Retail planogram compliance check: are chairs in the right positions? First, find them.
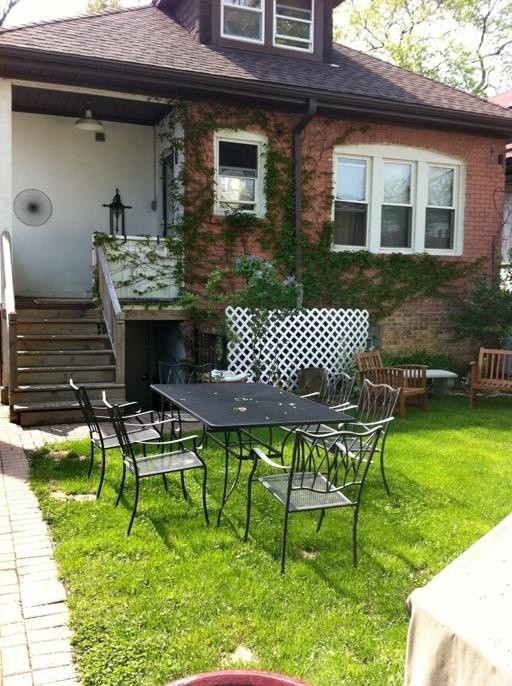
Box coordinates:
[279,369,354,472]
[356,352,429,419]
[69,379,168,499]
[158,361,212,449]
[296,379,401,496]
[100,391,209,536]
[243,426,380,574]
[469,347,512,407]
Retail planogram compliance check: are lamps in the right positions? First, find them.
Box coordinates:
[74,99,105,129]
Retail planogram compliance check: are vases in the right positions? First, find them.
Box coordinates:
[464,386,469,393]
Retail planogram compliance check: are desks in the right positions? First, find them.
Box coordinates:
[151,384,355,527]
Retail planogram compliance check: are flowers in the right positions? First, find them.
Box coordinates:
[460,370,473,385]
